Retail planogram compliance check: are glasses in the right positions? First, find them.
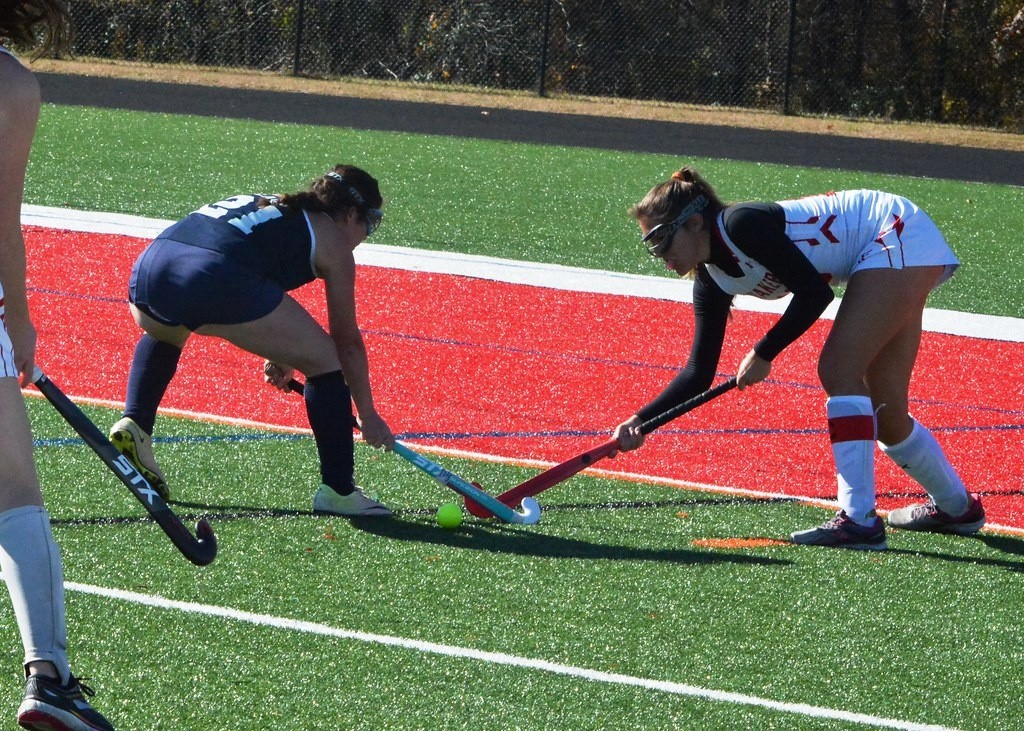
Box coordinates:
[364,210,383,235]
[641,222,676,258]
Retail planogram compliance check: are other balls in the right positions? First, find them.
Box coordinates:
[435,502,463,528]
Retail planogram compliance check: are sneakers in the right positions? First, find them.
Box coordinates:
[107,417,169,502]
[789,510,887,551]
[313,484,393,518]
[17,672,115,731]
[887,492,985,532]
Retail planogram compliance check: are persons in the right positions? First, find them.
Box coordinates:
[108,163,398,517]
[610,164,986,551]
[1,0,117,730]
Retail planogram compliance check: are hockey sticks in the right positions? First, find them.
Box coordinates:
[265,363,541,525]
[30,365,217,567]
[465,376,737,518]
